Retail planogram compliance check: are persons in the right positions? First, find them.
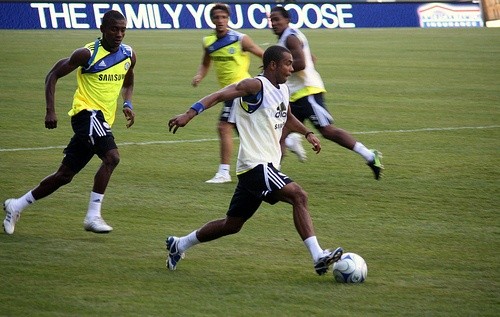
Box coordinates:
[2,10,137,236]
[165,3,385,276]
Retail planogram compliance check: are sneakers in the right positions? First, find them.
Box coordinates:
[287,132,307,162]
[366,148,385,180]
[83,216,114,233]
[2,198,21,234]
[205,170,232,184]
[313,246,344,276]
[165,235,182,271]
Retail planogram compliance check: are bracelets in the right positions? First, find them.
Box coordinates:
[190,101,206,115]
[122,100,133,110]
[305,130,314,139]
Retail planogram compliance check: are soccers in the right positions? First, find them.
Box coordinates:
[333,252,368,283]
